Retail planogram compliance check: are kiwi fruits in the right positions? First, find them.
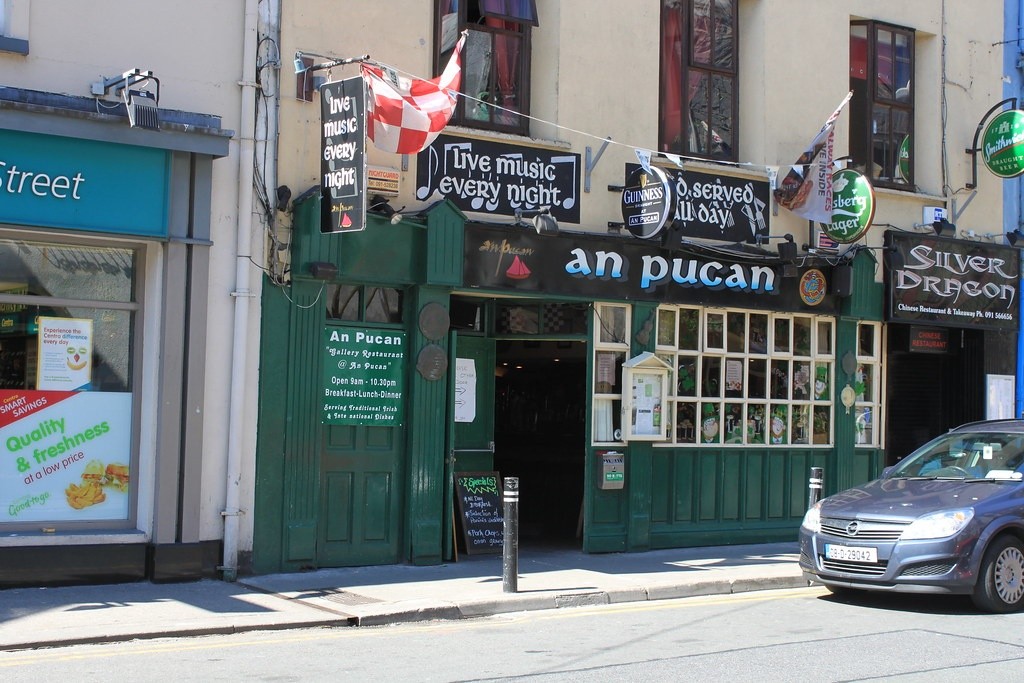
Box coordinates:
[67,347,87,353]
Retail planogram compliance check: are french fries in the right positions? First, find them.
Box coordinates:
[63,483,105,509]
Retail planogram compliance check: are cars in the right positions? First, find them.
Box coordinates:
[678,331,809,402]
[799,420,1024,614]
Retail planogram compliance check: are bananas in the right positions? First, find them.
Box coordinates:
[67,357,86,370]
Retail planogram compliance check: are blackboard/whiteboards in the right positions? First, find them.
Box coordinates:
[452,470,505,555]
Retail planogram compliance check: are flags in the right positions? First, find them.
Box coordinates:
[364,34,466,154]
[774,103,840,225]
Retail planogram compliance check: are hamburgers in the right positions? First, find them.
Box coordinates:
[80,461,129,491]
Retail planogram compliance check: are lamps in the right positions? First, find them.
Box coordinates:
[988,231,1017,247]
[914,218,957,238]
[656,218,686,251]
[104,69,162,130]
[370,196,402,224]
[514,208,560,236]
[855,243,905,271]
[755,234,798,261]
[309,262,338,281]
